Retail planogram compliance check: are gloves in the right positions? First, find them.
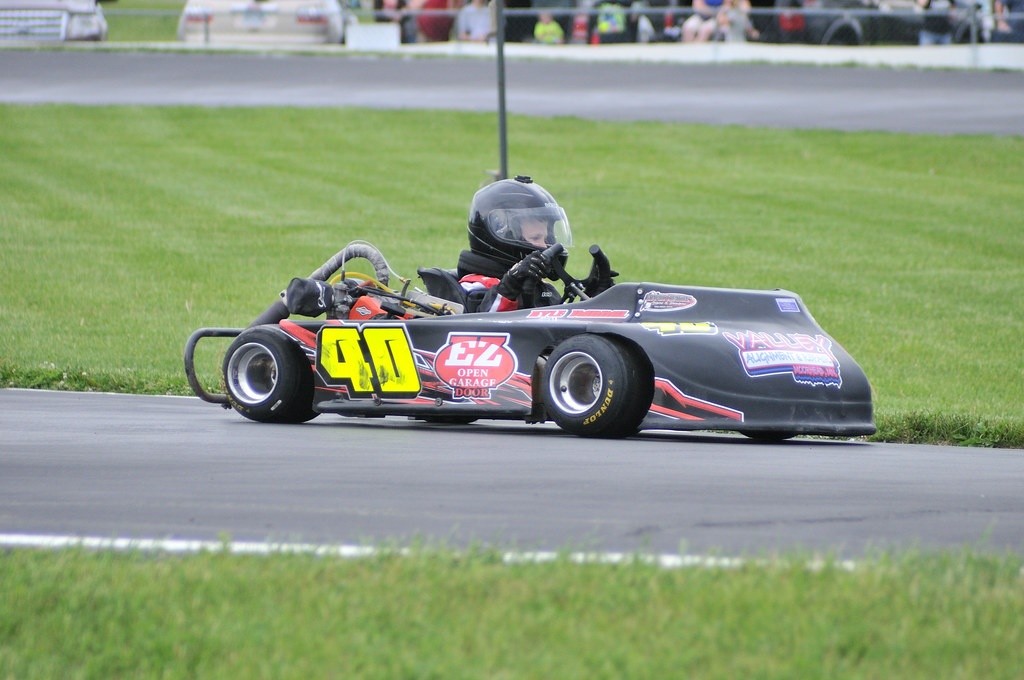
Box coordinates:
[496,250,550,301]
[610,270,619,287]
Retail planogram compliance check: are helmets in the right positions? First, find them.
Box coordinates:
[468,176,570,281]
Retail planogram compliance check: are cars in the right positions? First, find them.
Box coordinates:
[177,1,345,47]
[1,0,107,43]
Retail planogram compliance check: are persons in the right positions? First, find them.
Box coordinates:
[780,5,806,45]
[718,1,760,43]
[458,178,571,316]
[374,0,461,43]
[534,12,565,44]
[916,0,1013,45]
[457,0,506,44]
[680,0,726,43]
[628,0,685,42]
[594,0,631,44]
